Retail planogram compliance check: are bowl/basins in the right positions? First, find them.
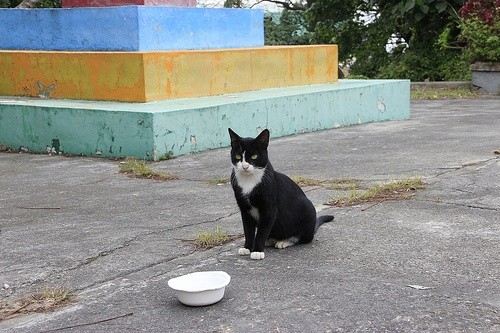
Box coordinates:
[167,270,231,306]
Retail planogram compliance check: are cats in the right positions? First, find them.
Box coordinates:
[228,127,335,259]
